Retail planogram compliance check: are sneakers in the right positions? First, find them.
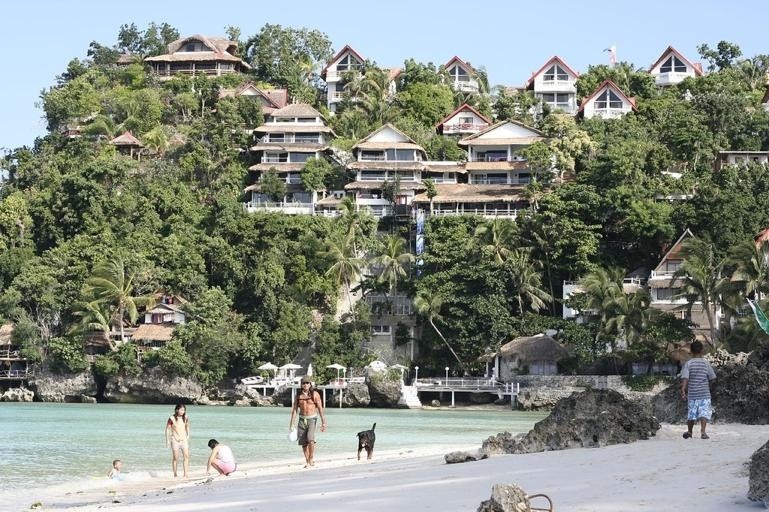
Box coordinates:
[683,431,693,438]
[701,433,709,439]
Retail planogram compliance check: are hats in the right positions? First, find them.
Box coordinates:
[302,376,310,384]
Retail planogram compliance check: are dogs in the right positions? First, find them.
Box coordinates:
[355,422,377,461]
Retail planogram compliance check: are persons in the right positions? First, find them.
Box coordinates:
[107,459,123,478]
[677,341,717,439]
[164,403,191,480]
[206,438,236,476]
[290,375,325,468]
